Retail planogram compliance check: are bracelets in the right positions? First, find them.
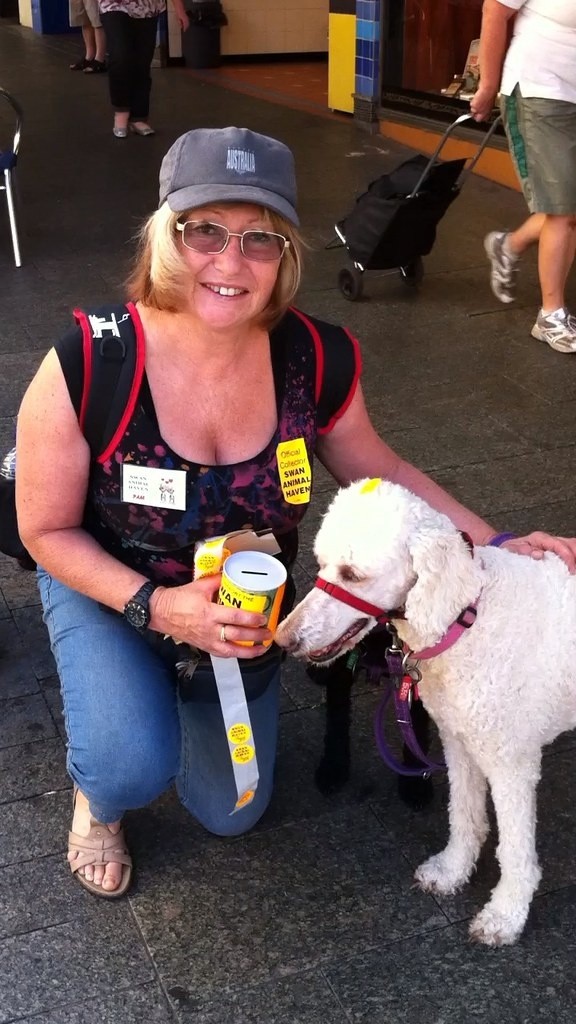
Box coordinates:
[489,533,515,546]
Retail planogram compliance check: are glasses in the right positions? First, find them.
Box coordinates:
[174,219,290,262]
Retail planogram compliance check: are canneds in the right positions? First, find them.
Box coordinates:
[216,551,287,652]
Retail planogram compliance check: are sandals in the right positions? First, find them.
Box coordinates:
[69,56,106,74]
[67,781,133,899]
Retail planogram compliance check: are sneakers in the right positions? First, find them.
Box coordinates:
[482,230,524,304]
[530,303,576,353]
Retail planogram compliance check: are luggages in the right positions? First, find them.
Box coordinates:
[322,107,501,302]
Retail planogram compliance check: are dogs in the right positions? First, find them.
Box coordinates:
[274,478,575,948]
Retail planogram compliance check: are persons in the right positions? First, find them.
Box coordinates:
[98,0,189,138]
[15,126,576,895]
[69,0,106,72]
[471,0,576,353]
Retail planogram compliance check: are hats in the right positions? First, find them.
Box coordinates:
[157,126,300,228]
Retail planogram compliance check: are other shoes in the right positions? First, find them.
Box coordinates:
[113,127,128,138]
[129,124,155,136]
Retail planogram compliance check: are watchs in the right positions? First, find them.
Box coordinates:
[124,583,156,635]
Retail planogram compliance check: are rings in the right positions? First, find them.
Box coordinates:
[220,624,226,643]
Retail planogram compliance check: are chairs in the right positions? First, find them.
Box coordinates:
[0,85,25,267]
